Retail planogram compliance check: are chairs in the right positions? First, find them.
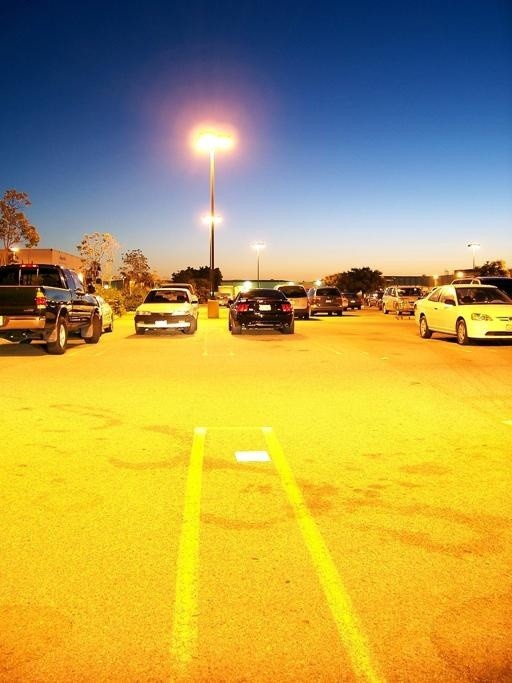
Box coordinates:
[461,290,486,302]
[153,295,185,302]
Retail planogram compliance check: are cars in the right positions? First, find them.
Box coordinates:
[229,288,295,336]
[307,286,342,316]
[95,294,114,334]
[135,281,199,336]
[365,291,385,309]
[341,293,361,311]
[413,277,512,345]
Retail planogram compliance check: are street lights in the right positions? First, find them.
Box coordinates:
[249,240,266,287]
[193,126,230,299]
[468,242,482,271]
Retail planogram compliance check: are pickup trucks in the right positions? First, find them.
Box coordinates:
[0,264,100,354]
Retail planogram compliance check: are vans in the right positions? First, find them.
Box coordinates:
[275,283,309,320]
[382,284,424,316]
